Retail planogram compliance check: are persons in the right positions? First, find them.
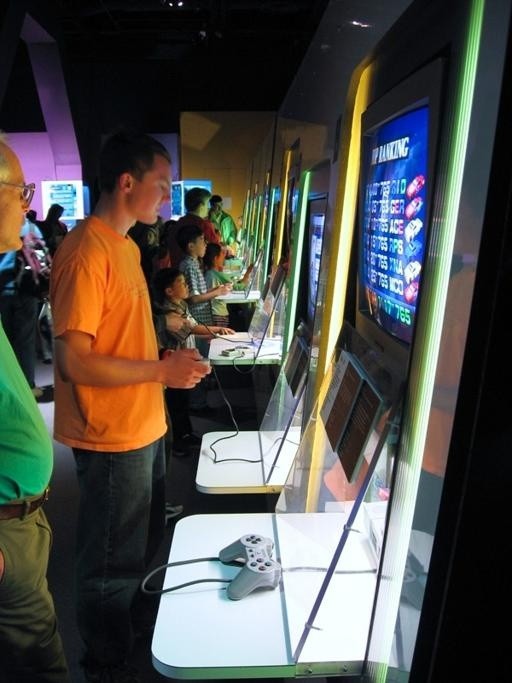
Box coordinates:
[0,139,72,682]
[49,126,211,681]
[1,185,263,454]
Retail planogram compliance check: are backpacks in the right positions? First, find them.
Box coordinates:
[15,223,55,300]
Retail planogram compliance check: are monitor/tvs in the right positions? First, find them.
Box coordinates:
[262,182,300,258]
[305,198,328,332]
[355,56,448,385]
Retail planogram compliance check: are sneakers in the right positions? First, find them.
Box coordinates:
[171,433,203,458]
[34,383,56,405]
[165,500,183,520]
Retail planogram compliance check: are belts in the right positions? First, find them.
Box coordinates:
[0,485,51,522]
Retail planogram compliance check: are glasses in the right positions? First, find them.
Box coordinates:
[1,181,36,206]
